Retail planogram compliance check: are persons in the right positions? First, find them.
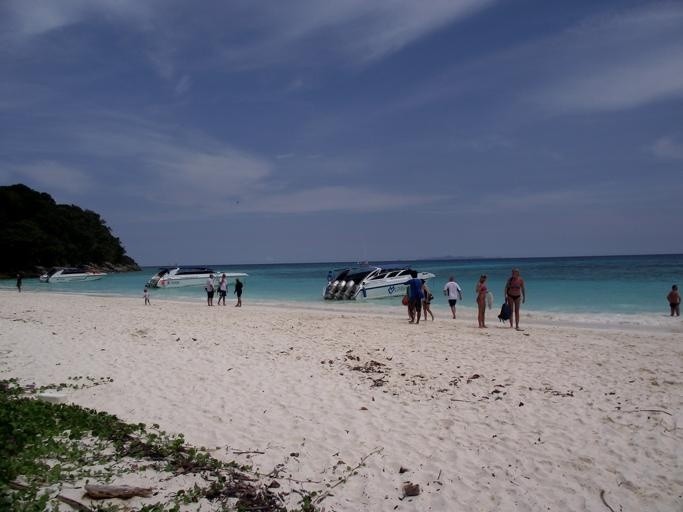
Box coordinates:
[15,273,22,292]
[666,284,681,316]
[404,270,425,323]
[420,279,434,321]
[406,287,416,320]
[206,274,215,305]
[141,287,149,304]
[443,276,462,319]
[233,278,242,306]
[216,273,228,306]
[504,269,525,328]
[326,270,333,285]
[475,273,489,328]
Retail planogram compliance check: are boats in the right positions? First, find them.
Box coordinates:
[143,264,252,289]
[320,265,438,306]
[39,266,109,284]
